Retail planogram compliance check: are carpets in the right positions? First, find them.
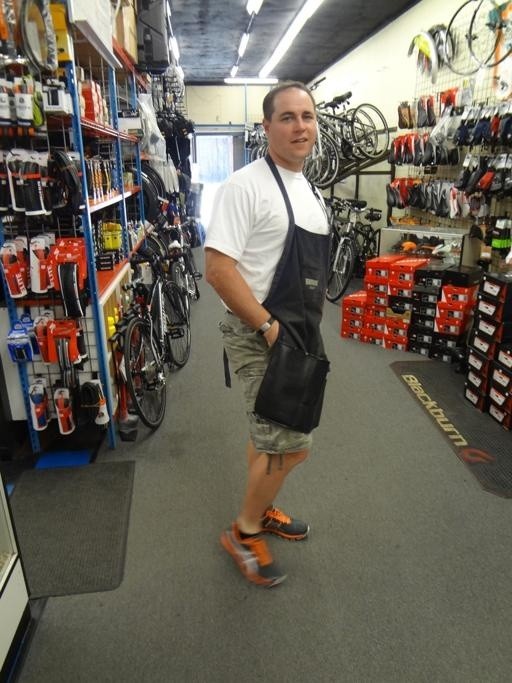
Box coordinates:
[384,355,511,498]
[8,460,138,600]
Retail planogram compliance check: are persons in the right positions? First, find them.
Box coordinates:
[202,81,334,588]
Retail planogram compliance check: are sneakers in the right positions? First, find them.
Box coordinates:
[218,520,287,588]
[260,503,311,540]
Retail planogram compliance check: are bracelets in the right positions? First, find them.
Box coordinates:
[259,313,275,336]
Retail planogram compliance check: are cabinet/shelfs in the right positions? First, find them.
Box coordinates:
[1,0,191,455]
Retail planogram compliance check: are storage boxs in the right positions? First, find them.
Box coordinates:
[339,255,511,430]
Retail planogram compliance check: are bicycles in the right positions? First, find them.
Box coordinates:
[322,194,382,302]
[123,221,202,429]
[244,79,389,188]
